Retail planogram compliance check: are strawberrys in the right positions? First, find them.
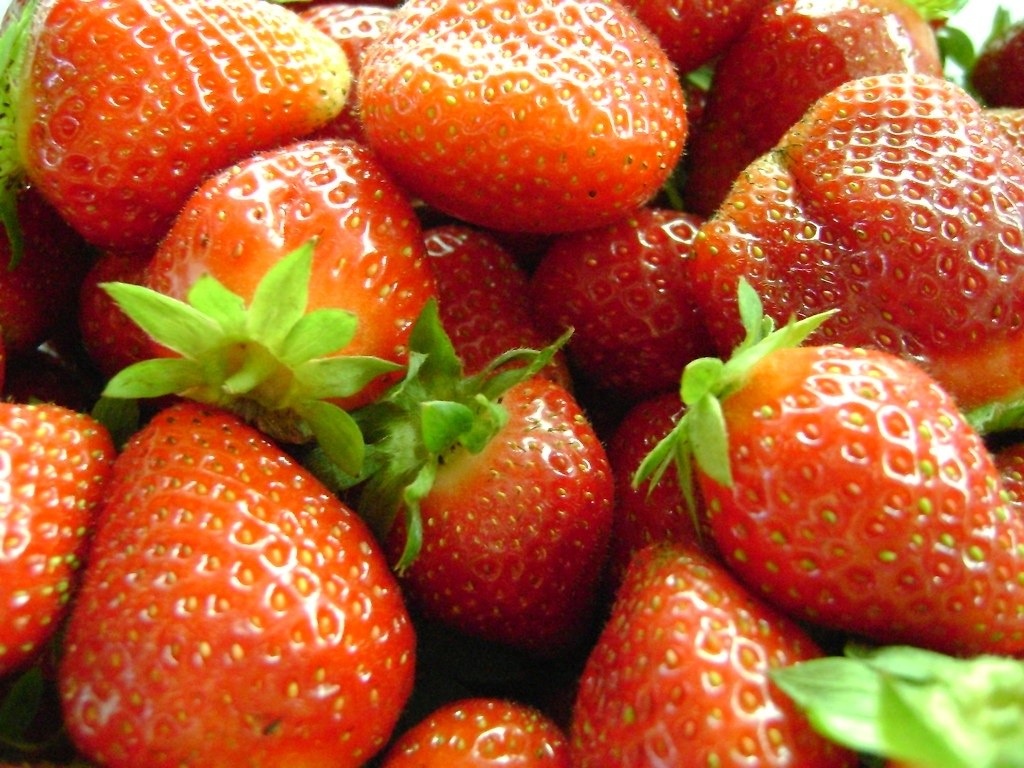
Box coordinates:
[0,0,1024,768]
